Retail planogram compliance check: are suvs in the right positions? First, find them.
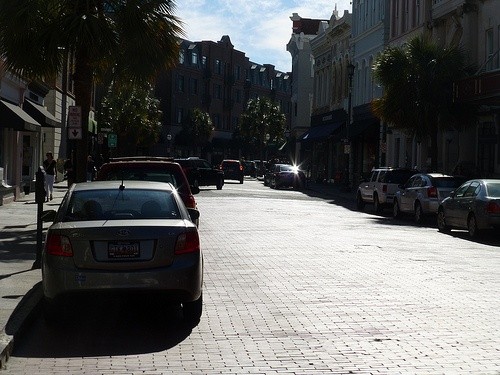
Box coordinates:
[219,159,247,184]
[90,155,201,229]
[173,156,225,191]
[354,165,419,215]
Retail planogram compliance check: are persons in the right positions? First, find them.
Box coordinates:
[88,156,95,182]
[368,158,378,173]
[241,157,247,169]
[269,157,277,167]
[99,154,104,164]
[55,158,63,182]
[43,151,58,202]
[250,162,257,178]
[279,157,288,163]
[421,152,438,173]
[405,159,412,169]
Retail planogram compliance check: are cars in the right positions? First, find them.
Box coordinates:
[391,172,469,227]
[436,178,500,240]
[40,178,205,328]
[241,157,306,191]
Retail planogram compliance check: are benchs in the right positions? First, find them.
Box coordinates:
[79,201,161,215]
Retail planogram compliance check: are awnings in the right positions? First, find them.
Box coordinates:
[24,98,61,127]
[297,120,346,142]
[0,100,41,131]
[334,115,380,146]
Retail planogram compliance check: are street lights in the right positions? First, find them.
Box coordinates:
[166,134,172,157]
[344,61,356,193]
[265,133,270,169]
